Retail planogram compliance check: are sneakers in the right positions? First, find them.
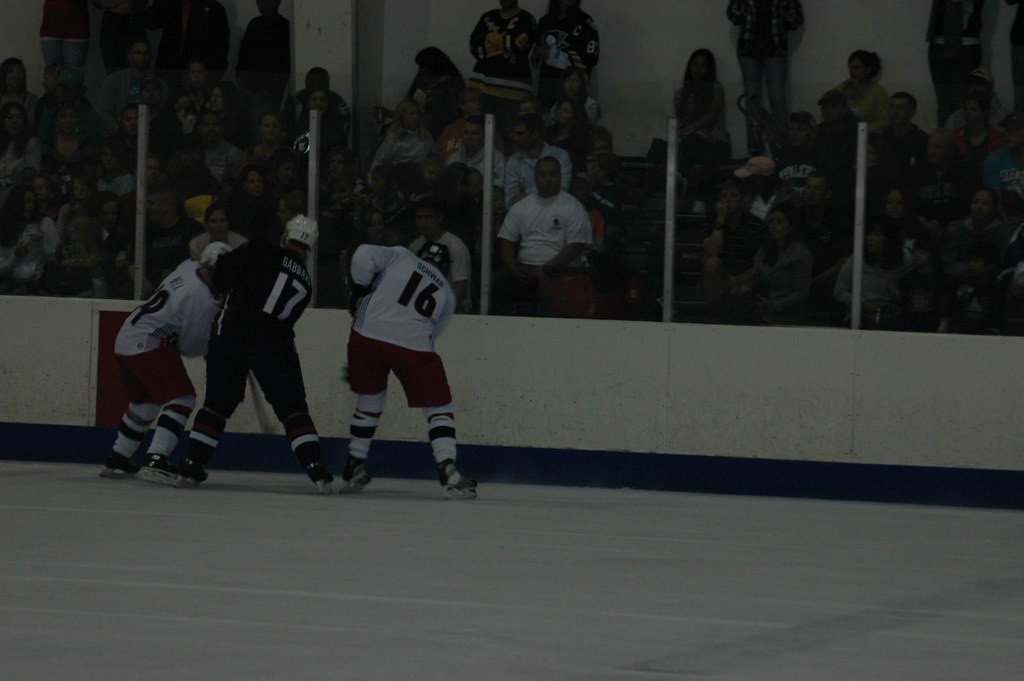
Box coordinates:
[99,448,129,479]
[134,453,177,485]
[303,458,334,496]
[339,453,373,493]
[437,458,478,499]
[173,458,208,488]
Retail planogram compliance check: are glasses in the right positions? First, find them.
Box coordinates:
[415,214,433,220]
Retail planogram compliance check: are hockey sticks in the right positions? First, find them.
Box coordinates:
[248,371,272,434]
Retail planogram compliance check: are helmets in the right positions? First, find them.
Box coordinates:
[280,214,319,252]
[200,241,234,268]
[417,240,450,270]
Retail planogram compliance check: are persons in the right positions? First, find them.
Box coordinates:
[91,0,151,75]
[406,203,472,313]
[925,0,984,128]
[674,48,731,203]
[234,0,290,82]
[186,204,248,261]
[530,0,600,109]
[726,0,805,164]
[177,214,335,494]
[465,0,537,126]
[0,36,1024,338]
[39,0,91,85]
[443,114,506,189]
[830,49,890,134]
[1004,0,1024,116]
[144,0,231,80]
[492,156,595,319]
[97,242,234,486]
[503,113,572,212]
[342,241,480,499]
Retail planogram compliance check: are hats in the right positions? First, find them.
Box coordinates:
[734,155,776,178]
